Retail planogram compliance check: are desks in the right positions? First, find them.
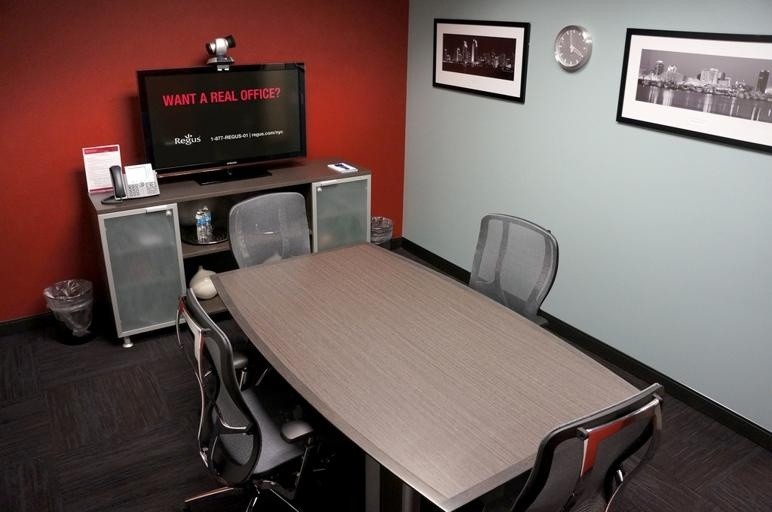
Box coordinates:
[211,241,643,512]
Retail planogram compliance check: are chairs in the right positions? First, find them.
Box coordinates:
[467,211,558,325]
[510,381,666,511]
[228,192,311,269]
[174,286,336,512]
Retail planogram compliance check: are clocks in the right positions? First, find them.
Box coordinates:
[552,24,593,71]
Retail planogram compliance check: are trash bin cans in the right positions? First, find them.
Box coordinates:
[43,278,95,346]
[371,216,393,251]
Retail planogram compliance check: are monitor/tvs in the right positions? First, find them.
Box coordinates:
[136,60,307,186]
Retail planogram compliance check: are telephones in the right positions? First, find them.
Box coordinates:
[101,162,161,204]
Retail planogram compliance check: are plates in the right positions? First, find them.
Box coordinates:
[180,224,228,244]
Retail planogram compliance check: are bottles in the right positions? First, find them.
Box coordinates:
[195,208,205,240]
[203,206,214,236]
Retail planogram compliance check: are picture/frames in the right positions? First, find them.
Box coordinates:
[432,17,530,104]
[616,27,772,156]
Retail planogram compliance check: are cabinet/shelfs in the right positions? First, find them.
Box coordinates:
[85,156,374,348]
